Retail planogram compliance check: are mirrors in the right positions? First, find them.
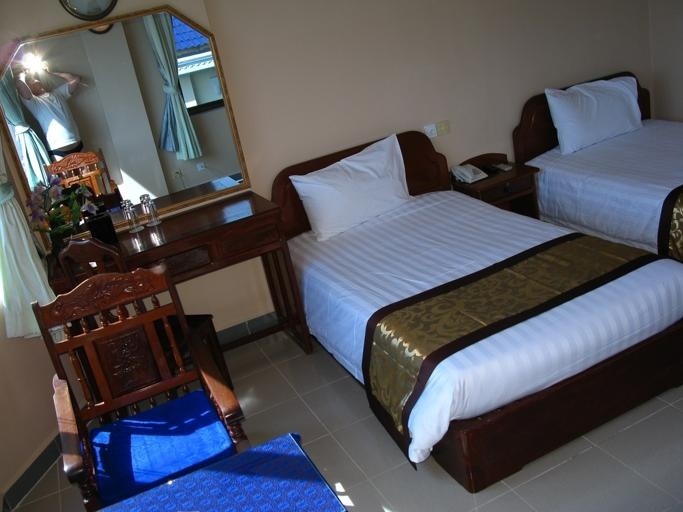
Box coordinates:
[1,6,254,261]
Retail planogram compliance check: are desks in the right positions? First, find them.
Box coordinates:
[43,191,313,425]
[106,170,244,224]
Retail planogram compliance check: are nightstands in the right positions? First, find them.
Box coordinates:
[451,147,541,221]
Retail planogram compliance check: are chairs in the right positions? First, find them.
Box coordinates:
[23,260,246,511]
[56,234,238,398]
[42,144,126,214]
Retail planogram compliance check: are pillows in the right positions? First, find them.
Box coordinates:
[541,75,639,156]
[287,131,413,242]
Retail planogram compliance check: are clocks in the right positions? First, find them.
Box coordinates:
[87,23,114,35]
[57,0,121,21]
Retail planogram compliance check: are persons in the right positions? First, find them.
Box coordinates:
[13,62,85,161]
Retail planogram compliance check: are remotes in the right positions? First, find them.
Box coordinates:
[493,163,512,171]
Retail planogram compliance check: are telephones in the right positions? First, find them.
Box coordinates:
[451,163,489,184]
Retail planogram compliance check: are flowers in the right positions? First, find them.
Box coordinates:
[26,175,101,237]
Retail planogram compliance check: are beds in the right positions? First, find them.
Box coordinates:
[266,126,681,493]
[511,71,682,259]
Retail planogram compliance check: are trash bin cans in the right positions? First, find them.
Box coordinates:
[147,314,235,401]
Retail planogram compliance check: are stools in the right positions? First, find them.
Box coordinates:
[97,431,349,510]
